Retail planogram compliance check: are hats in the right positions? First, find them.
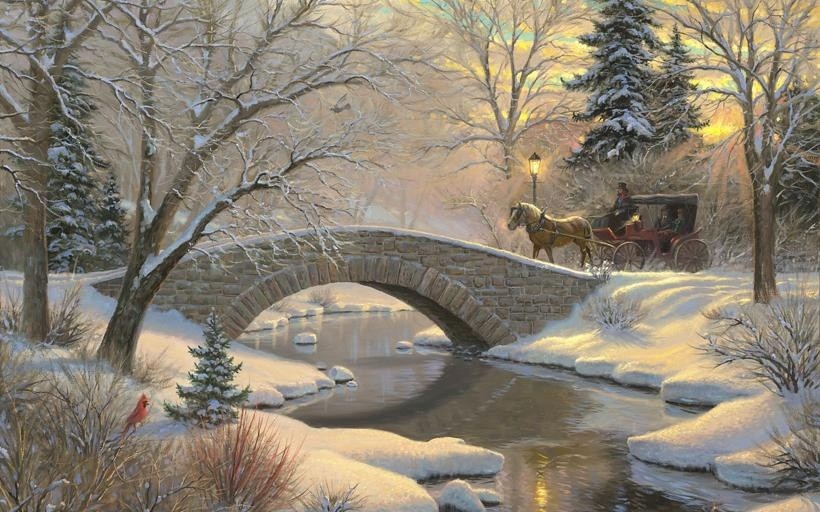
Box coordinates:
[616,181,626,189]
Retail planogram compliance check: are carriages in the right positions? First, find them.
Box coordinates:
[507,181,710,273]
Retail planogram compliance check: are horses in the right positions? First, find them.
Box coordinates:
[507,201,593,270]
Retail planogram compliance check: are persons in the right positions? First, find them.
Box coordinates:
[658,207,689,252]
[609,182,634,235]
[657,206,676,230]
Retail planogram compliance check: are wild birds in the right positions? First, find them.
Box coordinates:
[120,393,150,440]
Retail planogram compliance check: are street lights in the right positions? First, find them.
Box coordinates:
[528,151,541,206]
